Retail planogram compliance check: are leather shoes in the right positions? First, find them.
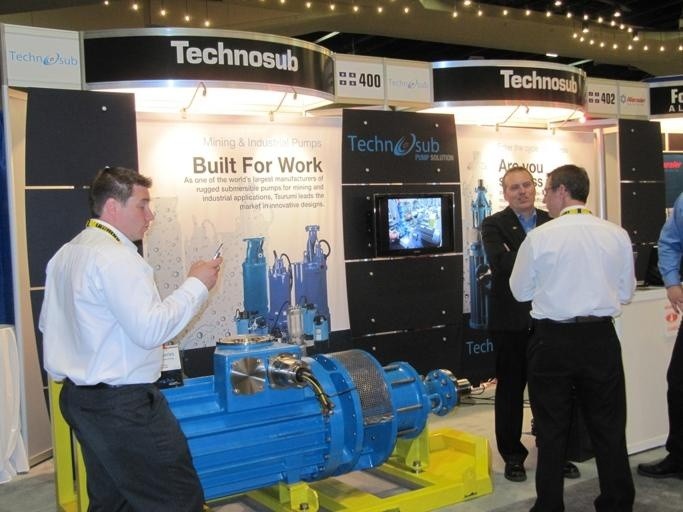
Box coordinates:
[503,464,527,482]
[637,460,683,479]
[563,462,580,480]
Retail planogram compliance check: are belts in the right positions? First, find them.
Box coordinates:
[67,378,127,390]
[550,316,611,324]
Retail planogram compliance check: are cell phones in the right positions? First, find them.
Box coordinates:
[213,244,223,261]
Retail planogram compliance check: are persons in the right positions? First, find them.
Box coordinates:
[481,167,581,482]
[638,192,682,479]
[510,164,637,511]
[39,165,223,512]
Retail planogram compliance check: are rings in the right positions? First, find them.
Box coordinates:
[216,266,220,271]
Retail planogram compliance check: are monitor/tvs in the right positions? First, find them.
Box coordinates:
[372,191,455,257]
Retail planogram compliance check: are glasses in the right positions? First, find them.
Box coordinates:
[542,185,560,196]
